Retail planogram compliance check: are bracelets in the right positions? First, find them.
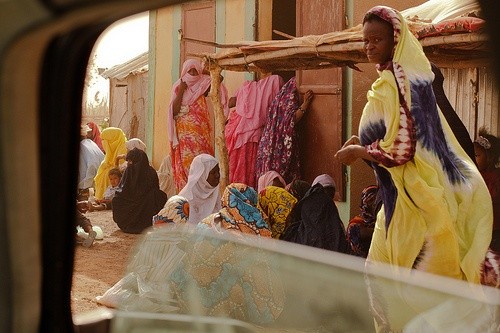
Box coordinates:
[299,106,305,115]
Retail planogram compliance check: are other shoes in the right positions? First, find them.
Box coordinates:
[74,234,86,244]
[83,231,97,248]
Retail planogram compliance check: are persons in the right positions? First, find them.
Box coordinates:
[77,122,382,258]
[169,57,224,196]
[223,75,313,189]
[472,127,500,252]
[334,6,493,285]
